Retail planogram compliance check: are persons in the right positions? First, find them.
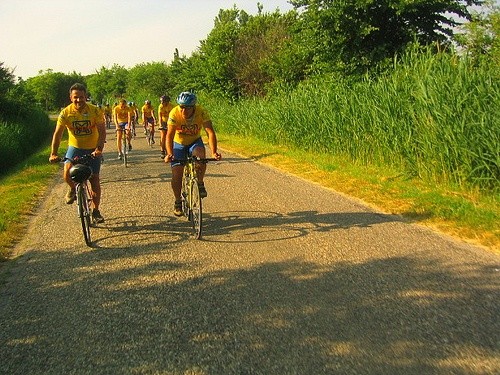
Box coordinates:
[142,100,157,144]
[112,102,117,124]
[164,92,221,217]
[99,105,104,113]
[128,101,137,136]
[104,103,112,124]
[158,96,177,159]
[114,99,132,160]
[132,102,139,124]
[49,83,106,223]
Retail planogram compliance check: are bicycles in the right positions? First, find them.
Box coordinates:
[161,151,220,240]
[115,126,131,168]
[106,114,167,158]
[59,152,102,245]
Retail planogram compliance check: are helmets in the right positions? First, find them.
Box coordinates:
[160,95,170,102]
[176,92,197,105]
[132,102,135,105]
[69,164,92,183]
[145,100,151,105]
[106,103,109,106]
[127,102,132,105]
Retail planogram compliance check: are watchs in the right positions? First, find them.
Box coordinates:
[96,146,103,151]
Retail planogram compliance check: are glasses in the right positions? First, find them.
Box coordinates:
[180,105,194,109]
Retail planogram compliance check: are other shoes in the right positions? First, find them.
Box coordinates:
[128,145,132,151]
[144,129,147,135]
[65,189,76,204]
[161,152,165,158]
[92,210,104,223]
[199,186,207,197]
[174,201,184,215]
[118,154,122,159]
[152,140,155,144]
